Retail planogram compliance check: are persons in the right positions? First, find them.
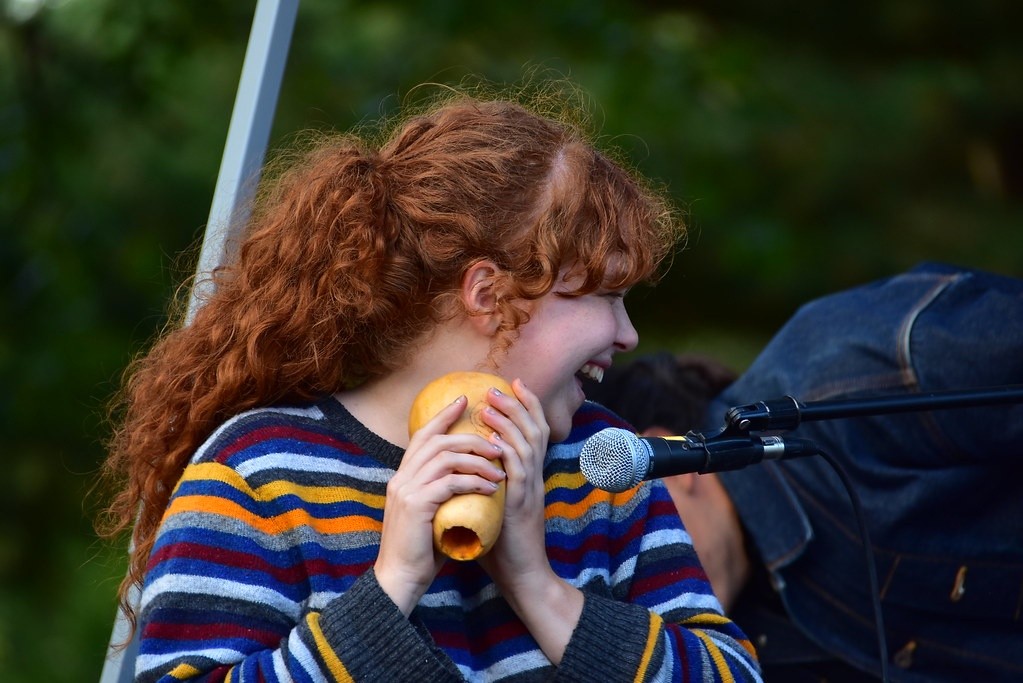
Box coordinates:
[584,263,1023,683]
[94,100,761,683]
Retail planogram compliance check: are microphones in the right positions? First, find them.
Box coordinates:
[579,426,822,493]
